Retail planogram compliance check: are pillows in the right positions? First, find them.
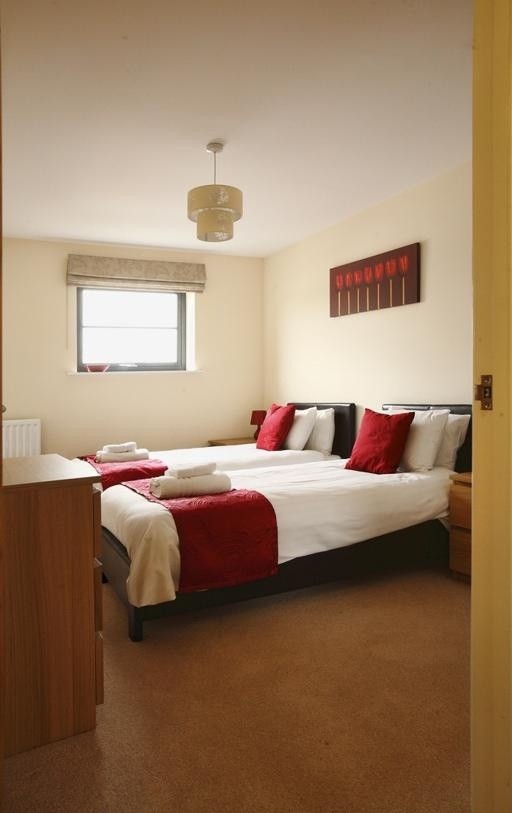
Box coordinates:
[433,412,468,472]
[376,409,450,473]
[305,408,335,457]
[284,408,316,450]
[255,403,294,450]
[344,407,418,475]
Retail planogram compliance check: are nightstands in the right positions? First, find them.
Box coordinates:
[207,436,258,446]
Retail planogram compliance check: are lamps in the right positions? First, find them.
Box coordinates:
[188,139,244,243]
[250,410,267,425]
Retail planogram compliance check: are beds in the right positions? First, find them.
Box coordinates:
[100,405,471,643]
[79,402,358,584]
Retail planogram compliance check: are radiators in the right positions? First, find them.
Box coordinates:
[4,419,41,457]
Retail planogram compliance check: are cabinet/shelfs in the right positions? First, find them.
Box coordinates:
[0,452,104,761]
